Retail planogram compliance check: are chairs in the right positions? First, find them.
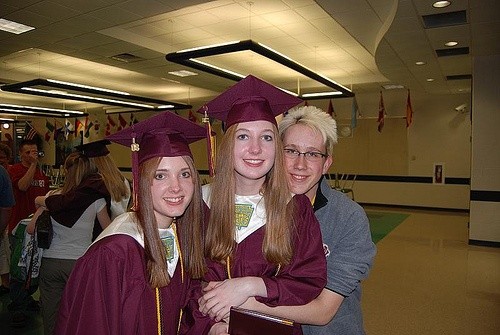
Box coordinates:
[328,169,357,202]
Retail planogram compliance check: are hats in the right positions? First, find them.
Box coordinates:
[197,75,304,178]
[102,110,217,212]
[73,139,112,158]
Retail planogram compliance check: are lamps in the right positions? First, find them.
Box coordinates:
[0,54,193,125]
[165,2,354,100]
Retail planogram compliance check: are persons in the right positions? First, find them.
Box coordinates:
[198,105,376,335]
[57,112,230,335]
[180,76,328,335]
[0,139,132,335]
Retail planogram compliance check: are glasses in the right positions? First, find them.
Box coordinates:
[283,148,330,161]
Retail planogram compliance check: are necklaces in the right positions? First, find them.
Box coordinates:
[155,222,183,335]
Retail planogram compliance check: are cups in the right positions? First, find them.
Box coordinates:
[51,176,61,186]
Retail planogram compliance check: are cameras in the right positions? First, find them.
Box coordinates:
[37,152,45,158]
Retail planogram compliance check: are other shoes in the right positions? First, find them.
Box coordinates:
[9,309,27,328]
[22,300,41,311]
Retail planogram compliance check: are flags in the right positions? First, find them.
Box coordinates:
[45,113,139,144]
[327,88,414,133]
[24,122,37,139]
[175,110,200,123]
